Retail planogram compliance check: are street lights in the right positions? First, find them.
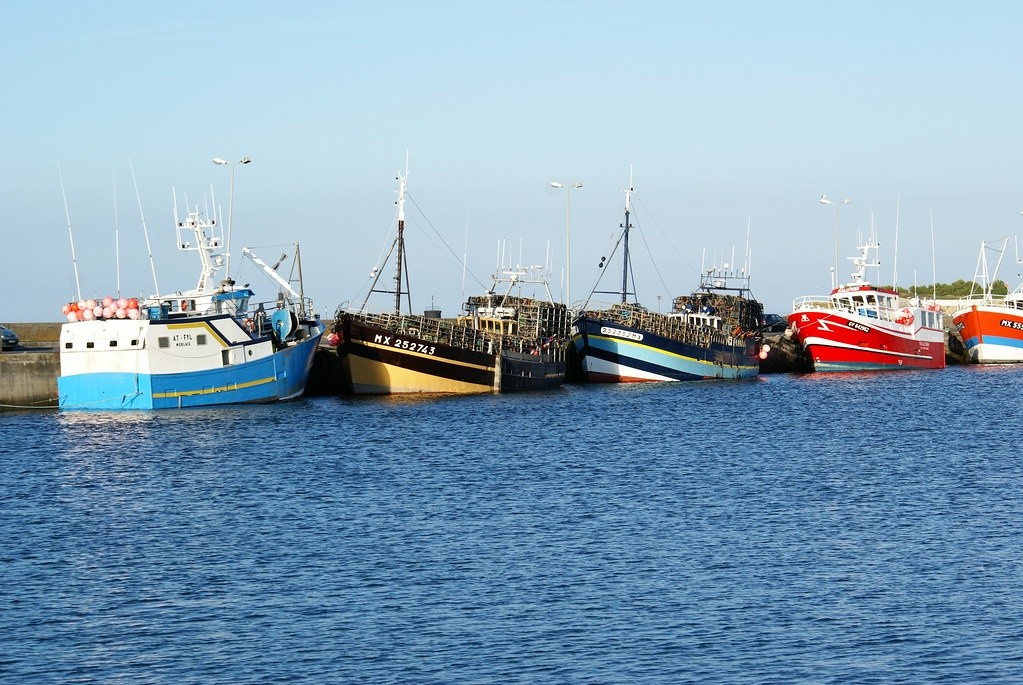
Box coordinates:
[818,198,852,290]
[548,182,584,312]
[209,156,252,281]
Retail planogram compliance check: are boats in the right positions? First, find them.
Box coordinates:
[326,148,575,397]
[567,162,764,382]
[57,156,327,413]
[782,195,948,373]
[951,237,1023,363]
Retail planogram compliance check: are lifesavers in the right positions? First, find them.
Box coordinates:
[948,336,963,354]
[243,318,254,329]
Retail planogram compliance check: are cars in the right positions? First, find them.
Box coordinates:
[760,314,788,332]
[0,324,19,349]
